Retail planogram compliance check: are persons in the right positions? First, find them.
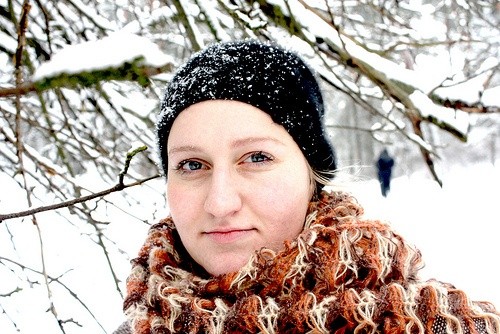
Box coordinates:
[375,145,395,198]
[108,38,497,334]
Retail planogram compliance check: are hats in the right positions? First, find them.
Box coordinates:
[156,38,338,194]
